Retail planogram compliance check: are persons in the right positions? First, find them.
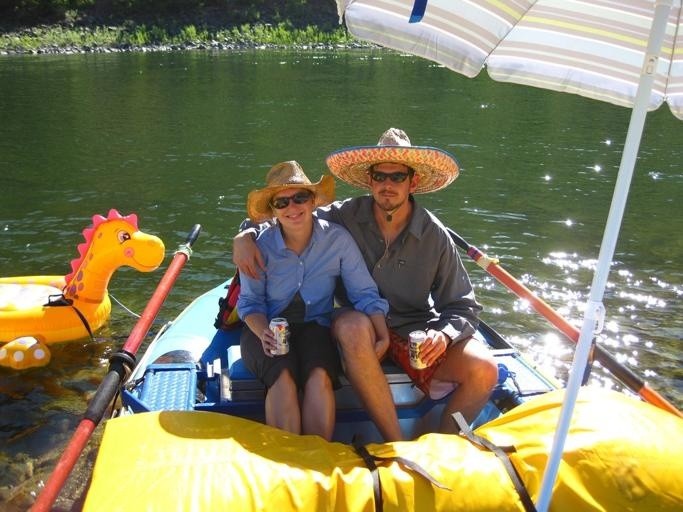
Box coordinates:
[237,188,390,441]
[232,163,499,442]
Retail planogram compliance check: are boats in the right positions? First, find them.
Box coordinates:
[110,254,560,511]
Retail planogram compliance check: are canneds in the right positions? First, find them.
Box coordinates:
[269,318,289,356]
[409,330,427,369]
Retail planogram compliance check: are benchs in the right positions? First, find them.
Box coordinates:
[214,316,488,418]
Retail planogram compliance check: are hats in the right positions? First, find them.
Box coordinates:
[246,160,336,225]
[325,128,460,196]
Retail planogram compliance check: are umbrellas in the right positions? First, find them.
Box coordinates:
[336,0,683,512]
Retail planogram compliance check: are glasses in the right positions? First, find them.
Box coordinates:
[268,191,310,210]
[370,171,408,183]
